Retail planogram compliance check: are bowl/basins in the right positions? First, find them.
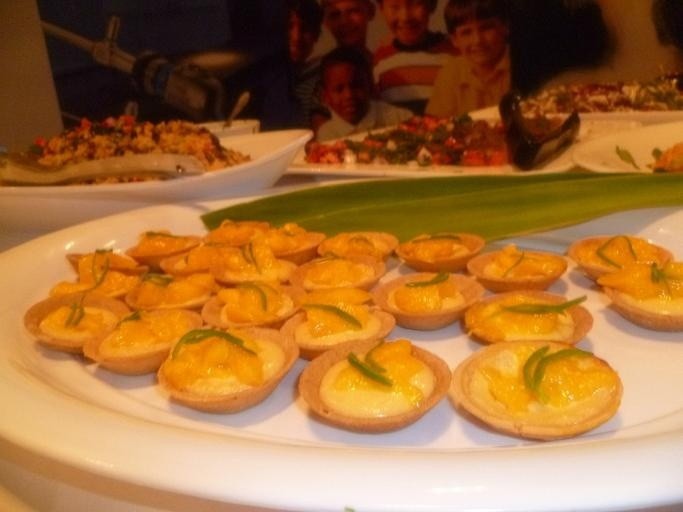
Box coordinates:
[0,128,314,239]
[199,120,262,139]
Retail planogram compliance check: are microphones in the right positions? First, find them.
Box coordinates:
[106,46,228,121]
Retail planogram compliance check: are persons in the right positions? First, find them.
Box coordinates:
[296,0,377,130]
[422,0,516,120]
[315,43,415,142]
[372,0,452,117]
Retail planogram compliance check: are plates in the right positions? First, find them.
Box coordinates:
[284,116,581,176]
[0,177,683,512]
[573,119,683,175]
